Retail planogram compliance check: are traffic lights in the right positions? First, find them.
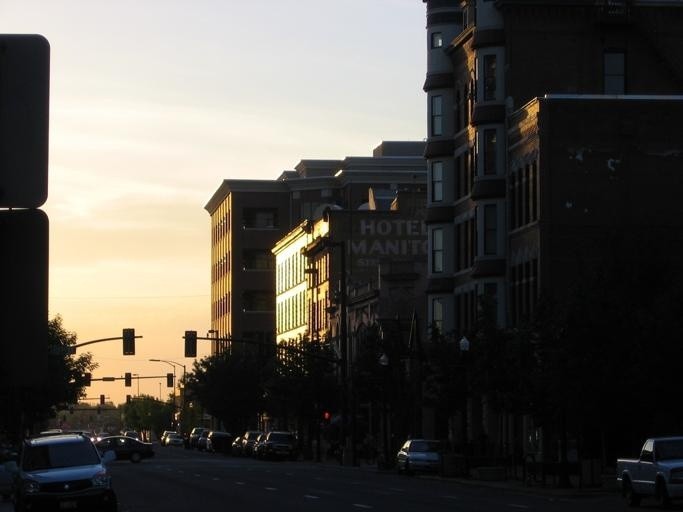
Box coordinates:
[323,409,330,424]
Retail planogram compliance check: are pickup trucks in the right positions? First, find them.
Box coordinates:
[616,437,682,509]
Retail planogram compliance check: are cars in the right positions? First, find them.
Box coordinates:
[8,431,116,512]
[397,440,445,479]
[3,426,155,464]
[159,426,305,462]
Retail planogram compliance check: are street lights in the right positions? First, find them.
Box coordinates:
[379,355,392,468]
[460,337,470,475]
[149,359,185,408]
